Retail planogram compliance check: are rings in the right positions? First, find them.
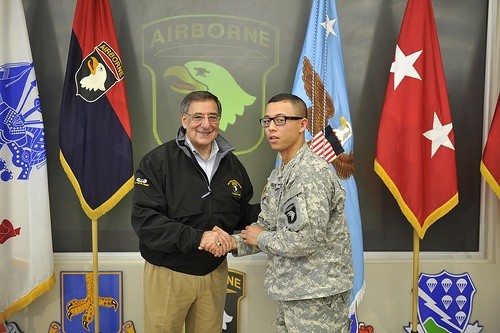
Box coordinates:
[216,242,221,247]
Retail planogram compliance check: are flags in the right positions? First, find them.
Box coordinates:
[372,0,459,239]
[0,0,57,331]
[274,0,366,319]
[478,92,500,203]
[57,0,136,218]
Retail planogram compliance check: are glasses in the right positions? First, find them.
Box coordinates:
[182,112,220,124]
[260,114,304,127]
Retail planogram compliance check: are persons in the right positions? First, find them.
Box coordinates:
[205,93,354,333]
[130,90,265,332]
